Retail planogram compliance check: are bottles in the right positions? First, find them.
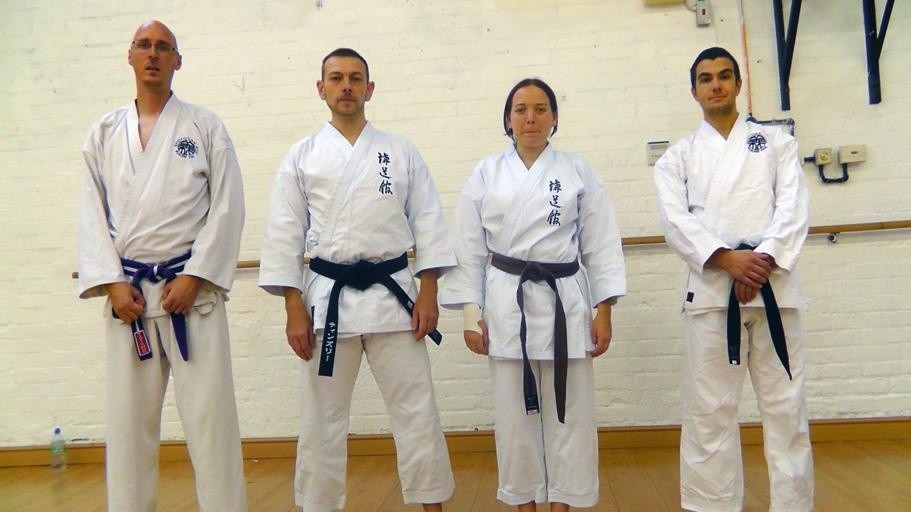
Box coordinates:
[50,427,67,472]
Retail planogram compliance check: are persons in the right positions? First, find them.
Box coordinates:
[654,46,815,512]
[75,17,246,512]
[256,47,458,511]
[439,76,628,512]
